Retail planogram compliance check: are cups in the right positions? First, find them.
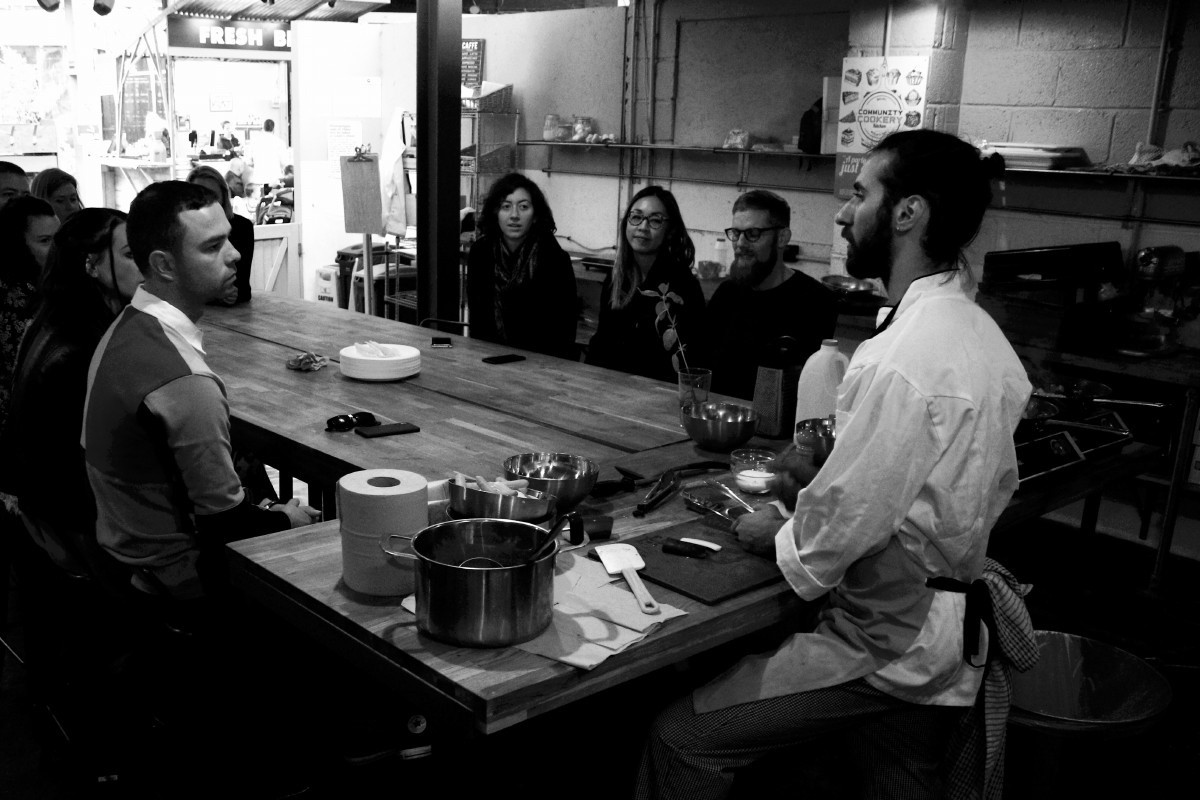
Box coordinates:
[730,449,777,494]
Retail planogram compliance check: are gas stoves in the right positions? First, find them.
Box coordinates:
[1012,410,1133,492]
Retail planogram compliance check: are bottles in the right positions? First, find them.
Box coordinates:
[793,338,850,452]
[542,114,592,141]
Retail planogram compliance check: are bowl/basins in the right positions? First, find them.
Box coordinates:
[503,451,599,509]
[446,478,556,521]
[683,401,764,450]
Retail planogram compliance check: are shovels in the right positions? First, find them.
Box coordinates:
[595,543,660,615]
[635,460,731,486]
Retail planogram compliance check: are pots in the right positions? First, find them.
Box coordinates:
[381,519,561,649]
[1022,398,1133,439]
[1029,376,1174,409]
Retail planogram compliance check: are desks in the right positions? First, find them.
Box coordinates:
[200,290,822,800]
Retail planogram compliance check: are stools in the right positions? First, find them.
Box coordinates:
[1,547,293,800]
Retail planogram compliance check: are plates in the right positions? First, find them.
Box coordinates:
[340,344,422,381]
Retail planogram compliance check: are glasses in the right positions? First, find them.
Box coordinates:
[725,227,785,242]
[627,211,670,229]
[325,412,380,432]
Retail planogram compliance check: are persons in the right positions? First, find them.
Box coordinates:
[705,190,839,410]
[467,172,579,358]
[0,160,31,207]
[247,119,285,168]
[631,127,1034,800]
[585,186,705,388]
[80,180,291,603]
[0,208,145,535]
[217,120,240,161]
[0,195,62,432]
[31,168,84,221]
[187,166,254,305]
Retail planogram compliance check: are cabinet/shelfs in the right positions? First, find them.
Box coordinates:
[380,110,522,336]
[571,268,1200,554]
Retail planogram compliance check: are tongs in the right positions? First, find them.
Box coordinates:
[679,475,756,529]
[637,470,682,512]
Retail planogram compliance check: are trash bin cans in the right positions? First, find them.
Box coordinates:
[988,630,1171,800]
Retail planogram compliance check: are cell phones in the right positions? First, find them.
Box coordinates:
[431,338,452,348]
[354,421,420,439]
[482,353,526,365]
